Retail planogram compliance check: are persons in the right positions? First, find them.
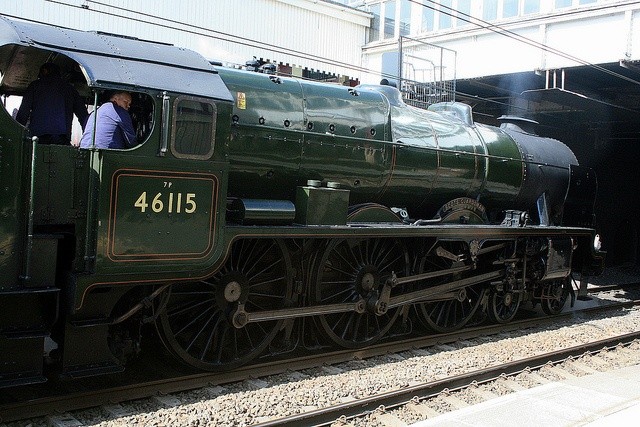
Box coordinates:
[15,62,90,147]
[80,91,138,150]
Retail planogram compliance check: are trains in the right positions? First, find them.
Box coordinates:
[0,12,596,386]
